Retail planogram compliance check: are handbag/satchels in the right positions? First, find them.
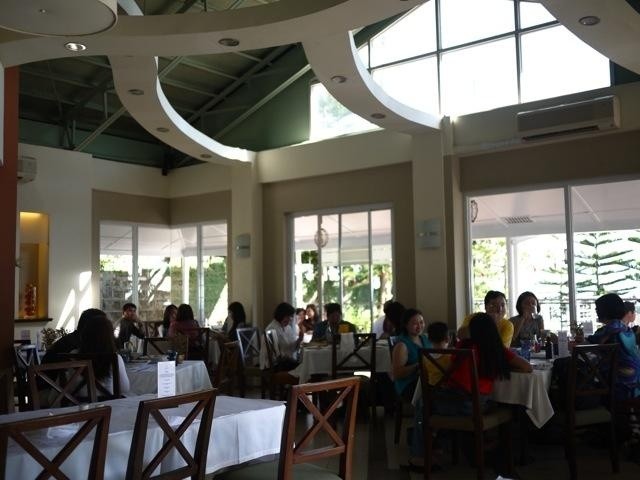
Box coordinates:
[551,356,603,410]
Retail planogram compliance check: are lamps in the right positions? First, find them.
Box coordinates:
[418,216,442,250]
[235,234,251,259]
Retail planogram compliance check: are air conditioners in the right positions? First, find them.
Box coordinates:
[17,156,38,184]
[515,94,620,144]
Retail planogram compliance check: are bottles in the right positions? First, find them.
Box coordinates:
[325,319,334,346]
[545,336,553,359]
[533,332,542,346]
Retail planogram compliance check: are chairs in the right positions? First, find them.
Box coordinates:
[417,345,522,479]
[3,321,638,414]
[387,336,424,445]
[2,403,113,480]
[211,374,362,479]
[538,335,624,478]
[122,388,219,479]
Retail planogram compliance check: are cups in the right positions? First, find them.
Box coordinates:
[338,323,350,336]
[520,340,531,362]
[530,304,539,318]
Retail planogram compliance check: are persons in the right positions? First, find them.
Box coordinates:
[260,301,406,410]
[42,309,132,406]
[392,292,639,474]
[120,301,246,361]
